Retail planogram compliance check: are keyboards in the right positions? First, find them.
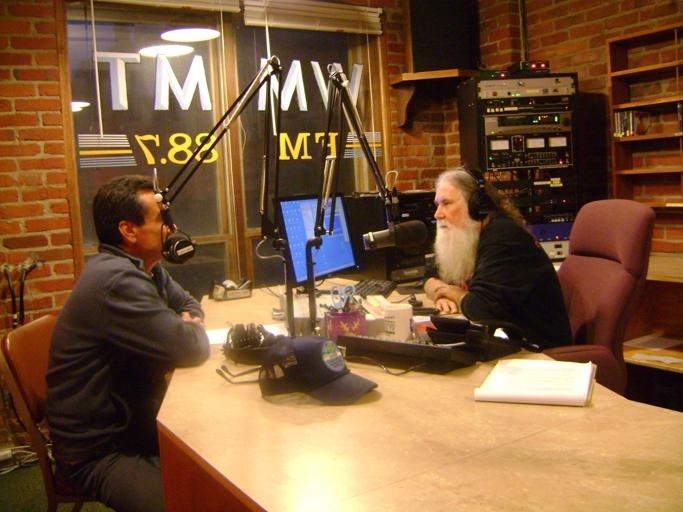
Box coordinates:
[344,279,397,299]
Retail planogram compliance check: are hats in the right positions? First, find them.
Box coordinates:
[259,335,378,405]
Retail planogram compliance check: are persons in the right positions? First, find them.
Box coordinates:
[419,165,573,351]
[42,174,212,510]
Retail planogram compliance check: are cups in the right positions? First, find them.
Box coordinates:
[383,302,412,340]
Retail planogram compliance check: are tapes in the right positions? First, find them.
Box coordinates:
[222,279,238,290]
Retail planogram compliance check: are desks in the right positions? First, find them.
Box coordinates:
[623,334,683,374]
[155,278,683,512]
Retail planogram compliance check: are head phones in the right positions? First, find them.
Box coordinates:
[157,201,195,265]
[462,166,488,221]
[223,323,277,365]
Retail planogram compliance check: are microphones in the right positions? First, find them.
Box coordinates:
[362,220,428,251]
[391,187,400,217]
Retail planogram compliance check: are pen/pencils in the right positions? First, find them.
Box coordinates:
[320,296,358,315]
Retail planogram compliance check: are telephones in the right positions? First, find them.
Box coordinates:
[426,316,521,362]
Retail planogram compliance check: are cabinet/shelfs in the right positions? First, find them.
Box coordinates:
[606,21,683,209]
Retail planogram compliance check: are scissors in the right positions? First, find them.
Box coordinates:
[331,285,355,314]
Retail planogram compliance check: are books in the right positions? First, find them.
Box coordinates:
[471,357,598,408]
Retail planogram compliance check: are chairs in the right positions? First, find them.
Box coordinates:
[542,199,655,397]
[0,314,97,512]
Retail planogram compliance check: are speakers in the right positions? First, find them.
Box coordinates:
[403,0,481,73]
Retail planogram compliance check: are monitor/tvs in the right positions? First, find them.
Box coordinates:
[272,192,360,298]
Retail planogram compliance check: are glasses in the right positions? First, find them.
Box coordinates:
[216,363,261,383]
